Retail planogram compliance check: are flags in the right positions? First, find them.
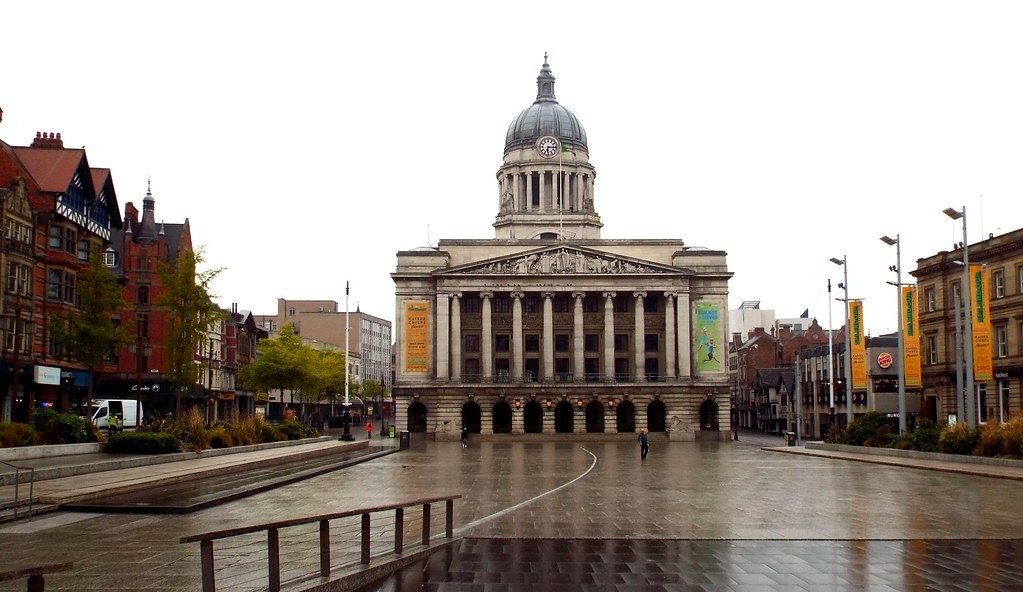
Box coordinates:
[562,145,576,156]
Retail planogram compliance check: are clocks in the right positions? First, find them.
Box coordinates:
[537,136,559,159]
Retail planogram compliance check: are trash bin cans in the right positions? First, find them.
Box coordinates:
[400,431,410,449]
[388,425,395,438]
[785,432,795,446]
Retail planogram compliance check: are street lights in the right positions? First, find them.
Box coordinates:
[201,341,221,430]
[881,234,907,437]
[127,322,153,427]
[830,255,853,423]
[0,288,38,422]
[730,376,739,441]
[380,380,386,436]
[942,206,975,430]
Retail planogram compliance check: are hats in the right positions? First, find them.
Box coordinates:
[464,428,466,430]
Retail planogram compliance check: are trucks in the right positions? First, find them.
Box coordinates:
[80,399,143,427]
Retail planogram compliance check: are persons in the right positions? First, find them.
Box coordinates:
[638,429,648,461]
[365,421,373,438]
[703,338,720,363]
[461,428,468,448]
[108,413,119,436]
[697,327,717,351]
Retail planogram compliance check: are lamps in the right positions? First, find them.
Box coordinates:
[515,399,521,410]
[607,397,614,410]
[546,399,552,410]
[577,398,583,411]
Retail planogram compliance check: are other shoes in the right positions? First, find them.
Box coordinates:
[641,457,647,461]
[462,444,467,448]
